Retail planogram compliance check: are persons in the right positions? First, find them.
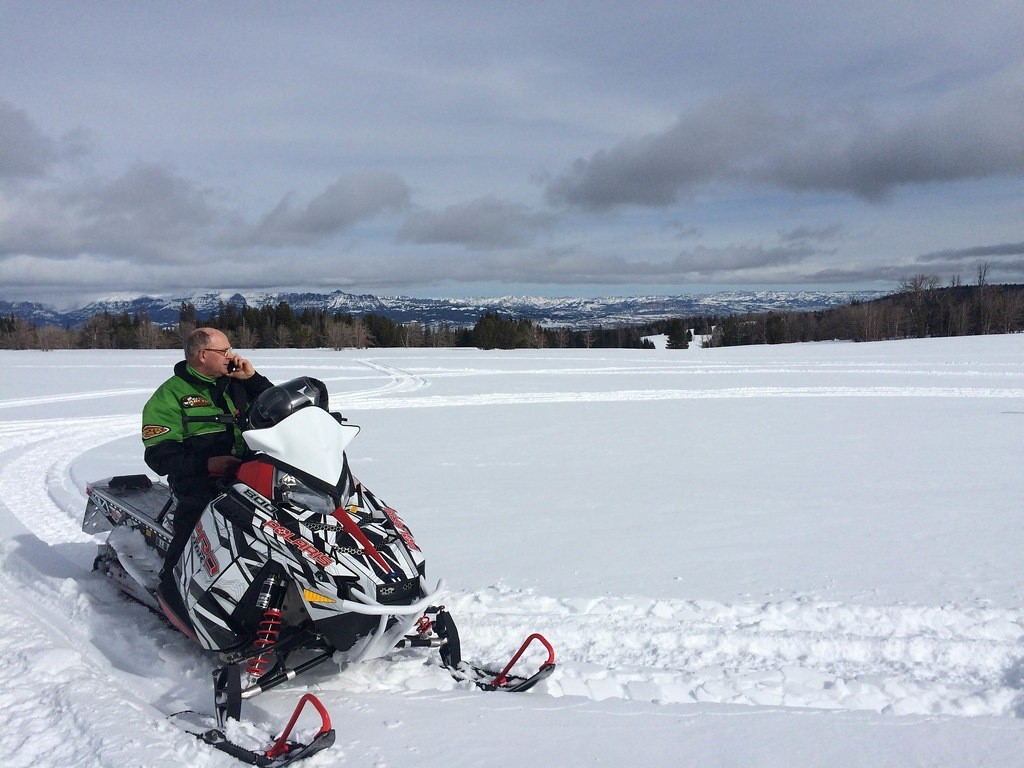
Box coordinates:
[142,327,275,589]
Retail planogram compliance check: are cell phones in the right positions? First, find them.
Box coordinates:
[227,361,234,374]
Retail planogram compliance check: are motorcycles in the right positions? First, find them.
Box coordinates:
[81,376,556,768]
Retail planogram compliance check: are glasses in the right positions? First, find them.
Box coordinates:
[198,347,232,356]
